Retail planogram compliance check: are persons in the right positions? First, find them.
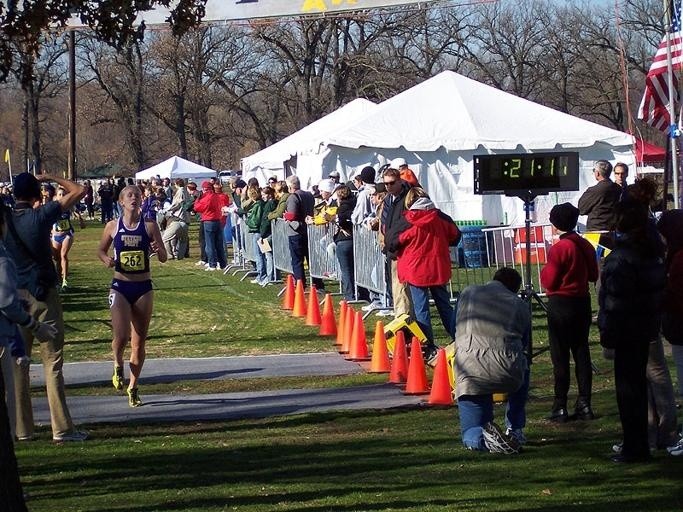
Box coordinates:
[160,216,189,260]
[98,182,114,224]
[213,180,229,259]
[400,168,423,189]
[381,168,412,318]
[328,171,341,194]
[194,181,228,271]
[234,180,247,196]
[304,179,340,225]
[0,173,92,444]
[450,267,534,455]
[210,177,216,185]
[596,199,660,463]
[282,175,327,296]
[164,178,192,258]
[96,186,168,408]
[1,210,57,512]
[229,176,240,207]
[128,174,162,190]
[79,180,95,220]
[268,177,277,189]
[162,178,173,201]
[52,187,75,289]
[353,175,363,189]
[33,182,54,209]
[384,187,462,348]
[350,167,376,225]
[179,183,208,265]
[114,176,127,217]
[234,186,266,284]
[323,186,358,305]
[248,177,259,186]
[265,181,290,220]
[141,186,157,220]
[365,184,392,317]
[656,208,683,457]
[577,158,622,231]
[613,189,681,454]
[257,186,278,286]
[1,184,17,211]
[107,178,120,218]
[539,202,599,422]
[611,162,629,193]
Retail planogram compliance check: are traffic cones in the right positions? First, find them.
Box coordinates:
[430,348,453,405]
[292,278,306,315]
[336,300,349,343]
[340,307,355,351]
[387,331,410,381]
[304,286,322,324]
[284,275,296,309]
[319,293,337,334]
[404,336,429,393]
[349,311,371,359]
[369,320,391,370]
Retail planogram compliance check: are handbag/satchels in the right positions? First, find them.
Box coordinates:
[6,216,62,288]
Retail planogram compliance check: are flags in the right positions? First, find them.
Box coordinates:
[638,1,683,137]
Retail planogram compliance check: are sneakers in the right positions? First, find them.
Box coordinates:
[205,267,215,272]
[665,433,683,456]
[362,302,379,312]
[53,427,89,441]
[376,309,393,316]
[505,427,528,444]
[196,260,206,266]
[17,433,41,441]
[126,387,143,407]
[62,280,68,288]
[478,419,525,458]
[112,362,125,391]
[612,441,626,451]
[217,267,222,271]
[258,278,273,286]
[250,277,260,283]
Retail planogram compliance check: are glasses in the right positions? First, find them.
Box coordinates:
[591,167,598,174]
[268,181,274,184]
[613,171,626,177]
[382,180,397,187]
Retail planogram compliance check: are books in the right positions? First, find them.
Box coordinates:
[256,238,272,253]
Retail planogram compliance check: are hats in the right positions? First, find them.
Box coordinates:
[361,167,375,183]
[201,181,211,188]
[549,202,579,233]
[318,178,336,192]
[391,158,405,170]
[328,170,340,178]
[232,180,246,189]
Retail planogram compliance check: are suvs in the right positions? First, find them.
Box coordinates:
[218,170,238,183]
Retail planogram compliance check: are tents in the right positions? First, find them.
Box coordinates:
[319,70,636,226]
[136,156,217,183]
[241,97,377,186]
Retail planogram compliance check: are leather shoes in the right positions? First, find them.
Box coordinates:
[611,454,651,464]
[575,405,593,419]
[542,408,568,422]
